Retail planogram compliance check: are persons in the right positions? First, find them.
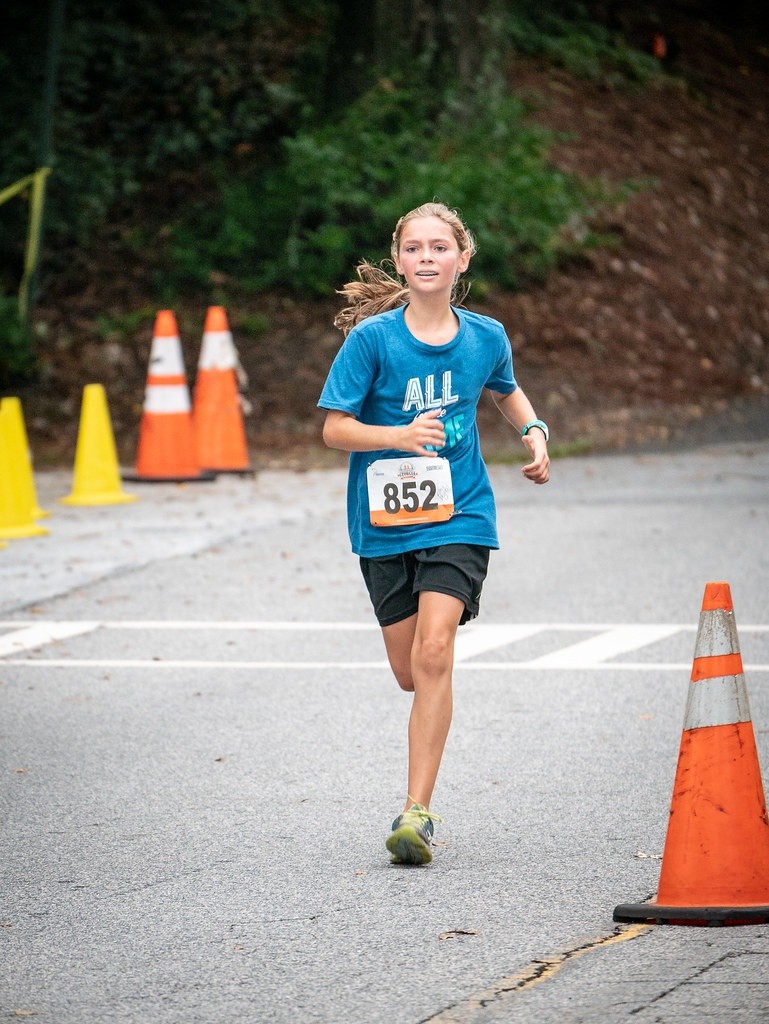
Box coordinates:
[314,200,549,867]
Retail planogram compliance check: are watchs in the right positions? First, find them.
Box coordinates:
[517,420,548,444]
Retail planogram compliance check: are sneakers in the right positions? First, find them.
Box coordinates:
[386,803,435,863]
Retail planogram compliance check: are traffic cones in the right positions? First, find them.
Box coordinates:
[0,397,52,543]
[120,311,214,481]
[63,381,138,507]
[608,580,769,927]
[191,302,251,475]
